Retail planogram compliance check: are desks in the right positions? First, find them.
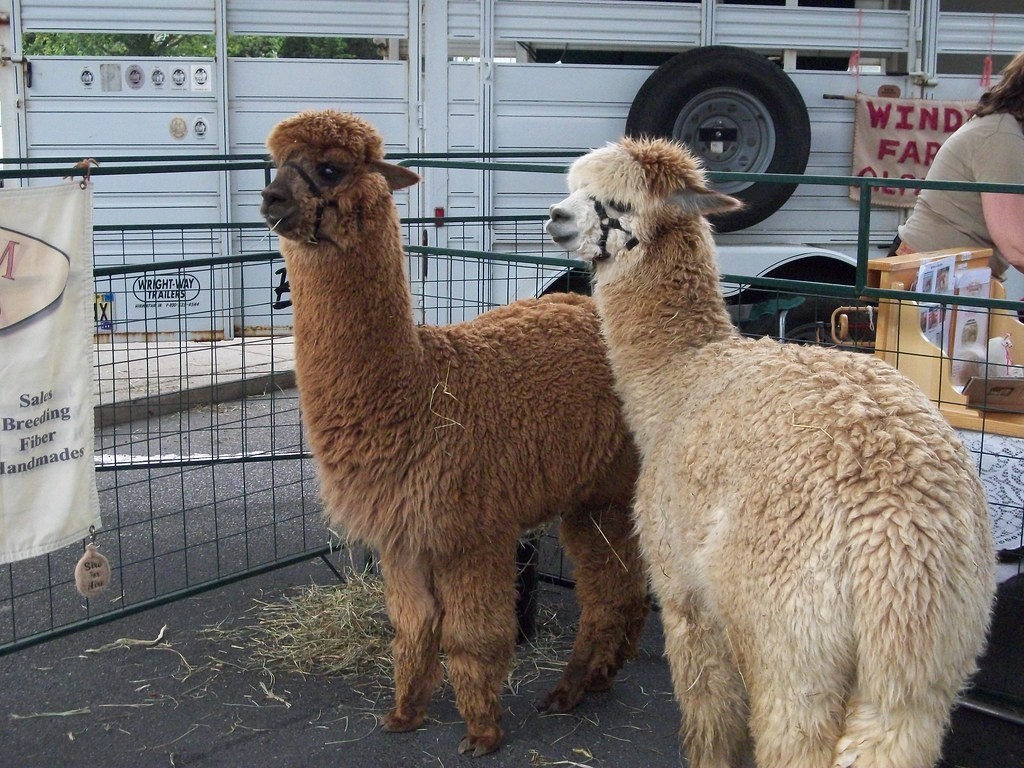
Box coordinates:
[829,247,1024,725]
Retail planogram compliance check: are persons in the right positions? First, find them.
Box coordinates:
[887,51,1024,277]
